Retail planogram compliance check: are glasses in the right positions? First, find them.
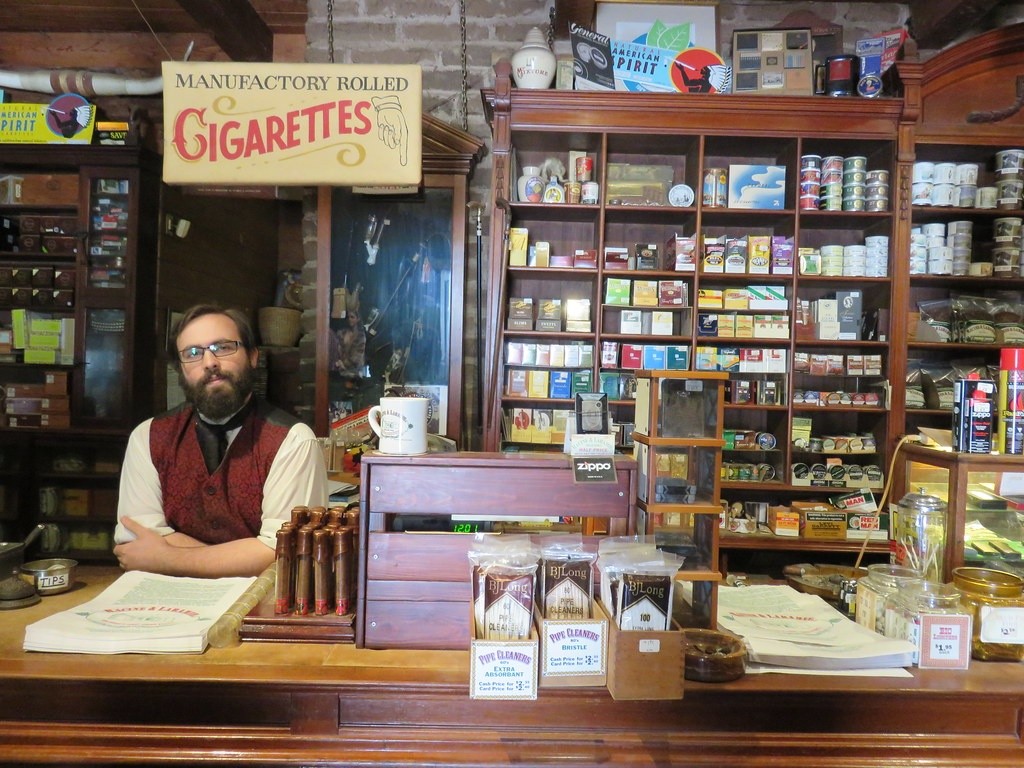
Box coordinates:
[176,341,244,363]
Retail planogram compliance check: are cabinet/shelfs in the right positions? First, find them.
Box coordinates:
[481,22,1024,555]
[0,106,163,566]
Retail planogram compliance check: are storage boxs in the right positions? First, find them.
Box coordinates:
[842,509,889,540]
[699,234,794,275]
[0,175,79,431]
[795,352,881,376]
[698,312,789,339]
[611,422,634,446]
[791,501,847,540]
[601,342,692,371]
[598,373,636,401]
[501,227,597,445]
[603,243,659,270]
[698,286,788,310]
[767,506,799,537]
[617,310,673,335]
[469,597,687,702]
[663,232,696,271]
[602,278,688,308]
[696,347,786,451]
[796,290,889,342]
[727,164,786,210]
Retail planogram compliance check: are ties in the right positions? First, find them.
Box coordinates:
[193,411,245,475]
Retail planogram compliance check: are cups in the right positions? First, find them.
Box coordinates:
[368,397,429,454]
[42,525,60,553]
[814,55,858,97]
[39,487,58,517]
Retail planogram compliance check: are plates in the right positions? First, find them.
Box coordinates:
[669,184,694,207]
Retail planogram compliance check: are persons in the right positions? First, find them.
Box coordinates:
[335,307,367,379]
[112,303,329,579]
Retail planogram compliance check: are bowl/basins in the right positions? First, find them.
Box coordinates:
[21,558,78,596]
